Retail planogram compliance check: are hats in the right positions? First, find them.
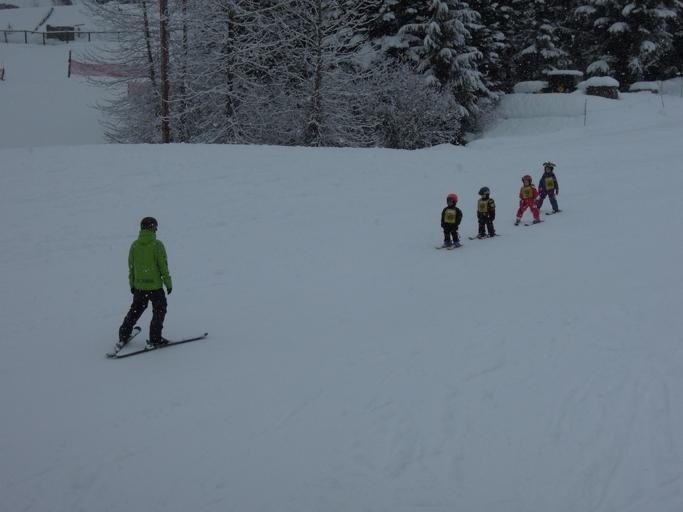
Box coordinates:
[140,217,157,230]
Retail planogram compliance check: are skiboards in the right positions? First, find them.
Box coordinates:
[435,242,462,250]
[105,326,208,359]
[468,233,502,240]
[514,220,545,226]
[545,210,563,215]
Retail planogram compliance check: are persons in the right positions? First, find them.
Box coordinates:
[117,216,172,346]
[515,174,541,223]
[440,193,462,246]
[475,186,496,237]
[537,160,559,212]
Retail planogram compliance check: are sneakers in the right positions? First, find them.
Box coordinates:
[552,209,558,213]
[149,338,169,346]
[477,232,494,238]
[442,242,460,248]
[514,219,540,225]
[119,334,131,342]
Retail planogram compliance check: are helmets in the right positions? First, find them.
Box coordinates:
[479,187,490,195]
[447,194,457,203]
[522,176,532,185]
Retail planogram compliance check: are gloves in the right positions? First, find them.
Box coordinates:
[167,288,172,295]
[131,288,134,294]
